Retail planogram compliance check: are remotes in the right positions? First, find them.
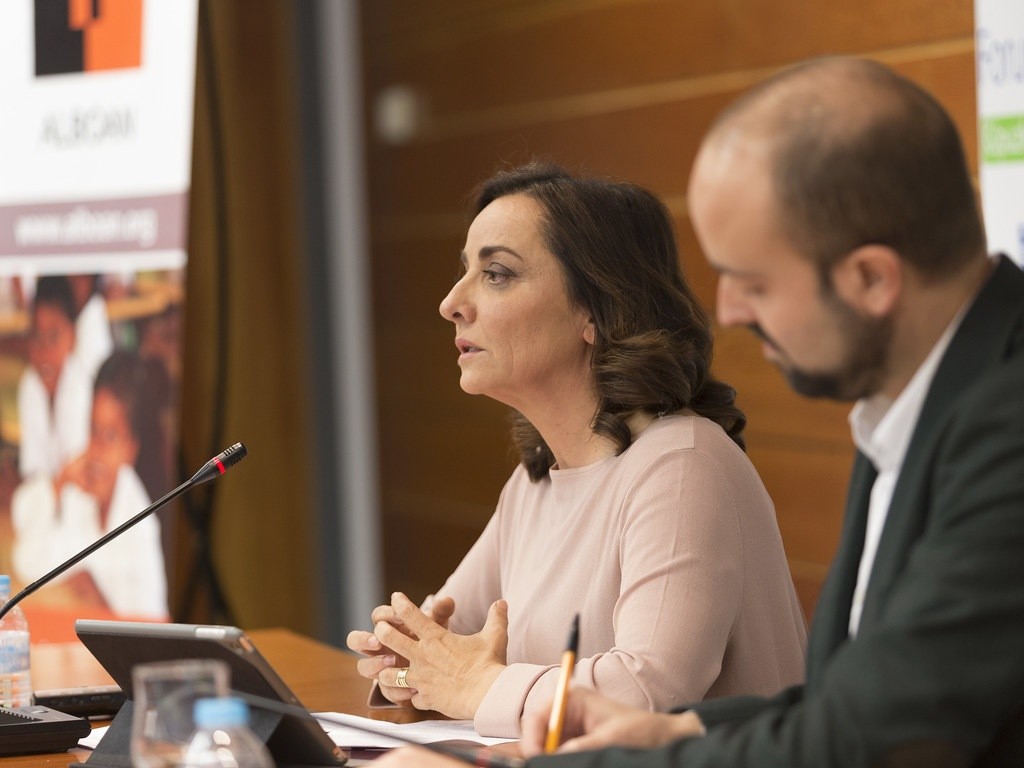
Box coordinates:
[33,683,127,718]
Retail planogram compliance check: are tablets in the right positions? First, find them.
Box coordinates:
[75,618,348,768]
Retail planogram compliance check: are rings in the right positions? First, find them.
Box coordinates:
[396,666,409,688]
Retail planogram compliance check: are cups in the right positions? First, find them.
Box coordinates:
[130,658,230,768]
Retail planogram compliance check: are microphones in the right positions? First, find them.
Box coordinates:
[0,442,249,758]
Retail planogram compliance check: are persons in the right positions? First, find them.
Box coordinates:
[346,161,809,754]
[359,56,1024,768]
[12,272,172,620]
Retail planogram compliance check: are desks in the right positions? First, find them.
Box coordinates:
[0,627,581,768]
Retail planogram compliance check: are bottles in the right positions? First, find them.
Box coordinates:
[178,696,276,768]
[0,575,31,708]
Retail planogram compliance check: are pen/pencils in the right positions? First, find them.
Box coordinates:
[542,611,581,754]
